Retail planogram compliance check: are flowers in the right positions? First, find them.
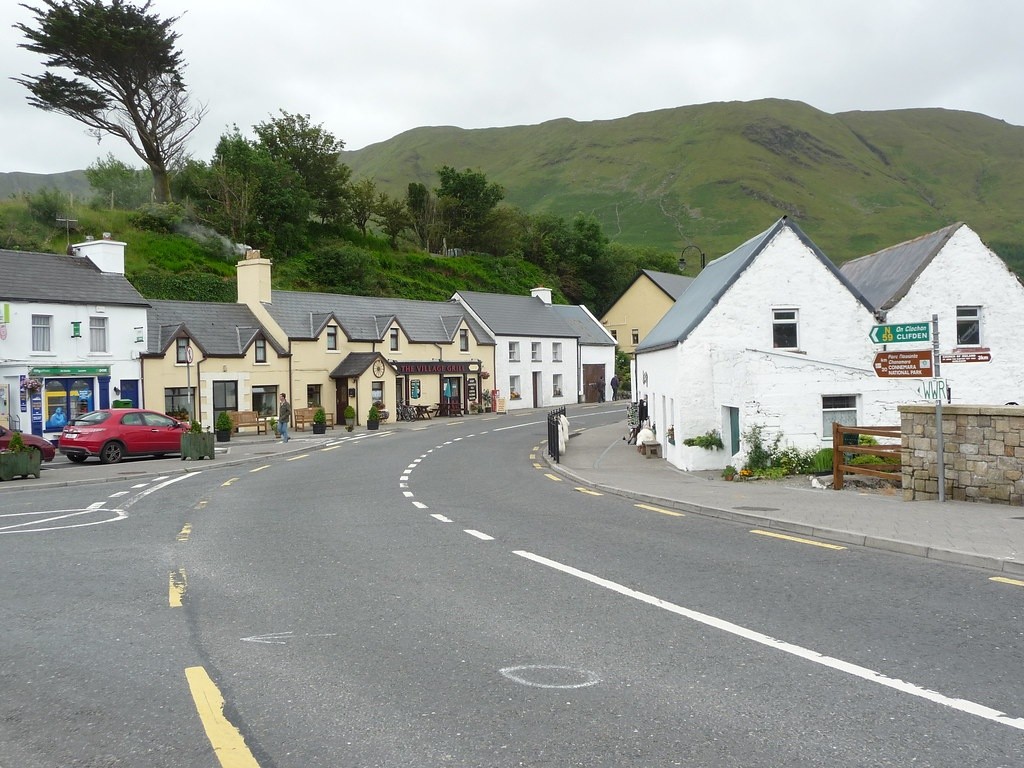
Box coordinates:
[21,378,43,399]
[739,469,752,479]
[479,372,490,377]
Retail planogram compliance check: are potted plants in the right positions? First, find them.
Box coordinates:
[344,406,355,425]
[313,409,326,434]
[367,406,380,430]
[721,464,738,481]
[471,401,479,415]
[215,412,233,441]
[0,432,41,481]
[483,389,491,412]
[811,448,834,477]
[273,424,281,438]
[180,421,216,460]
[478,406,483,412]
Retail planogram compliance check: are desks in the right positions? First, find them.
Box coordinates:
[414,405,433,419]
[432,403,466,417]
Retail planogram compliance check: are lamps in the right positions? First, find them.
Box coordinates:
[114,387,121,395]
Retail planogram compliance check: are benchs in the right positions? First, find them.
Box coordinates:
[294,408,335,432]
[228,411,267,436]
[641,440,662,459]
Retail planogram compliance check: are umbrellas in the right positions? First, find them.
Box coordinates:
[444,379,452,415]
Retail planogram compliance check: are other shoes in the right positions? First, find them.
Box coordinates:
[282,437,291,443]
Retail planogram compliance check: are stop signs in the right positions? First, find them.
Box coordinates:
[185,348,194,365]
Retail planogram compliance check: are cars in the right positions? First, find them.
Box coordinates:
[1,424,54,465]
[56,409,192,463]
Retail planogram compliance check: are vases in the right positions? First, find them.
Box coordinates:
[483,377,488,379]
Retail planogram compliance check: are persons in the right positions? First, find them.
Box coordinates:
[610,374,619,402]
[277,393,292,444]
[596,376,606,403]
